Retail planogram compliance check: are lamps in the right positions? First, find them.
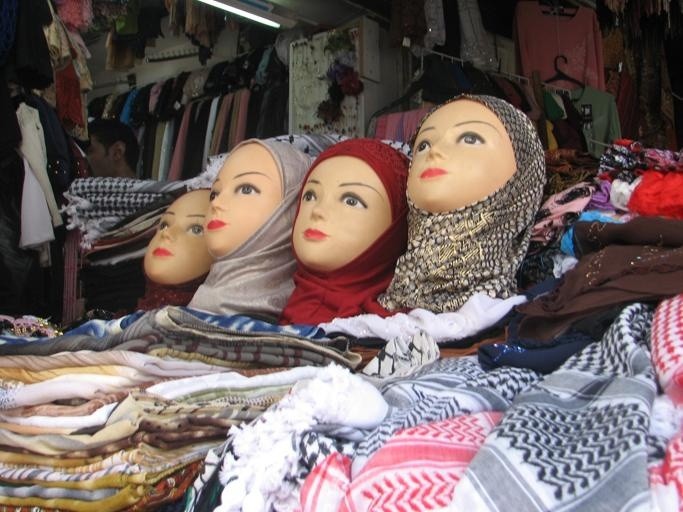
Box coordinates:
[201,0,300,39]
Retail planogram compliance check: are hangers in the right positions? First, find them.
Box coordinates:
[368,69,434,120]
[543,51,587,94]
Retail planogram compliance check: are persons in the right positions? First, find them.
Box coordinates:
[282,136,413,326]
[86,114,147,184]
[186,137,312,324]
[134,187,210,311]
[375,91,547,311]
